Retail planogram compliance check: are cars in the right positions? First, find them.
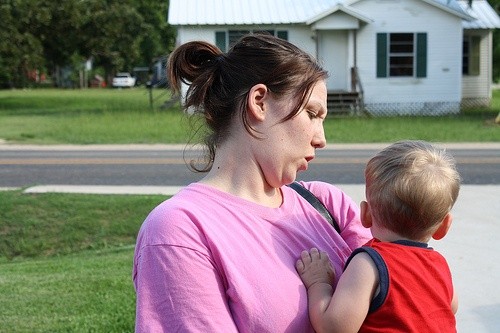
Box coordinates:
[112,72,136,88]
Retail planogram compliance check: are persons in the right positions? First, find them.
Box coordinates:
[132,33,374,333]
[295,140,461,333]
